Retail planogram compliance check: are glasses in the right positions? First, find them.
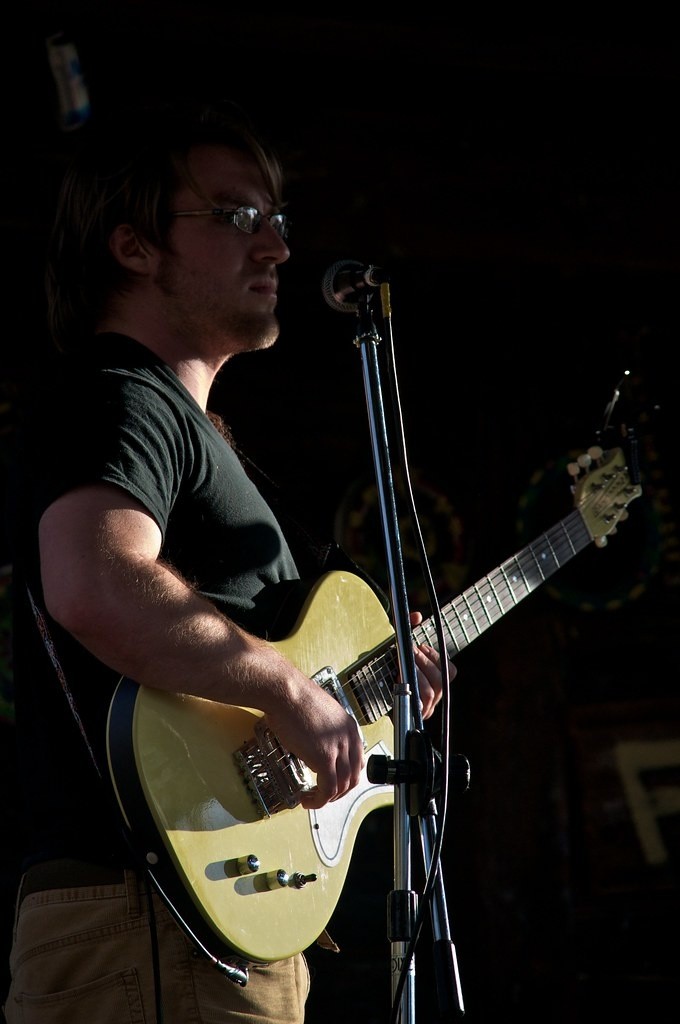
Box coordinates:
[173,205,292,241]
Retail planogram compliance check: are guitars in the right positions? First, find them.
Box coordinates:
[101,442,642,983]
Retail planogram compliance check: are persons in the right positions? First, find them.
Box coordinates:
[2,121,457,1024]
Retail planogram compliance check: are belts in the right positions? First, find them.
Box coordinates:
[19,859,148,907]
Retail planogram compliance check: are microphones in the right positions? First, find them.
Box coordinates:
[322,261,387,311]
